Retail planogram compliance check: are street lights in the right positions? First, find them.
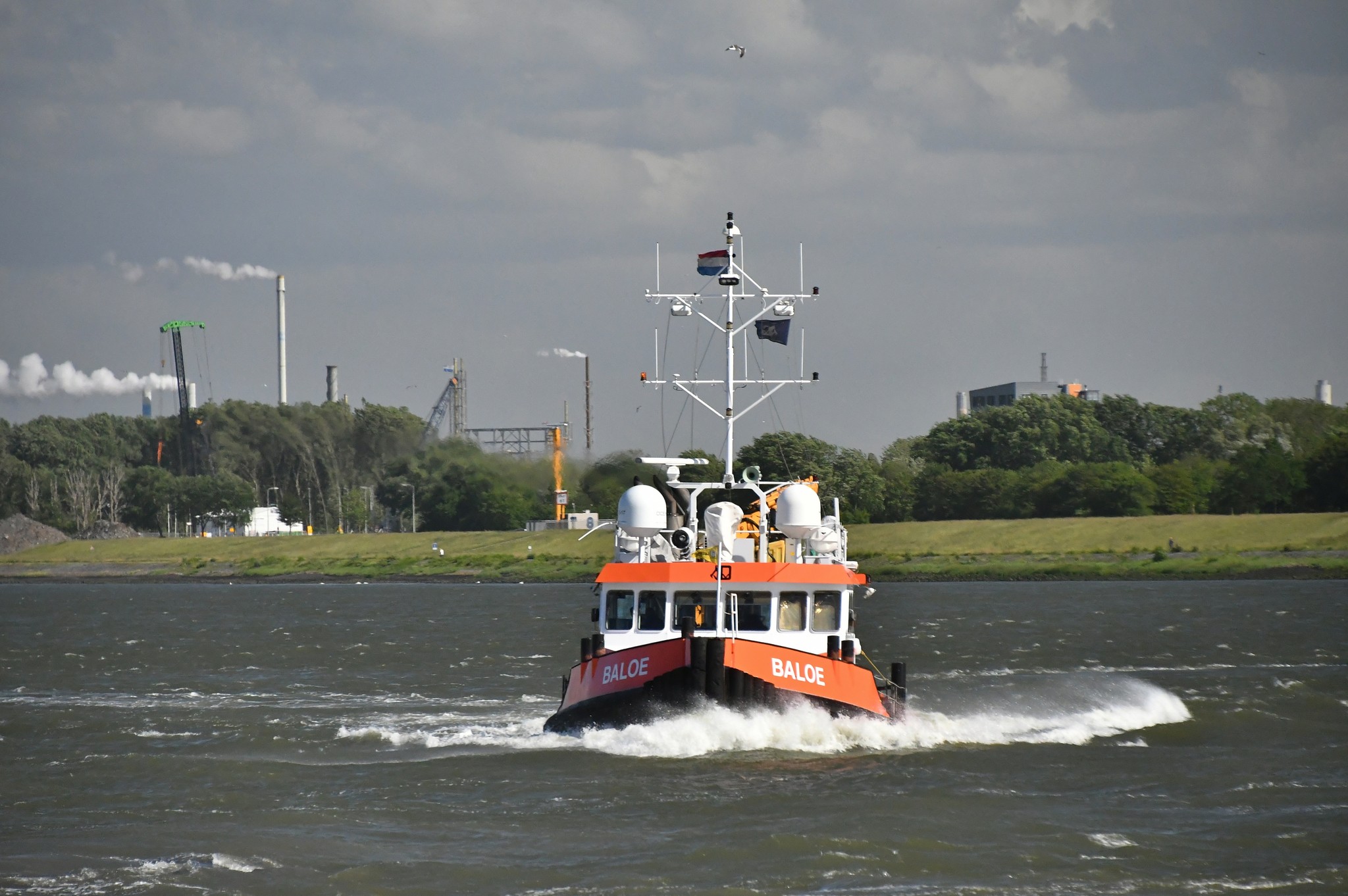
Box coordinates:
[401,483,416,534]
[360,486,373,511]
[267,487,279,536]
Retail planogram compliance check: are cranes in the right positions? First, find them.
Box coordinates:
[159,319,208,473]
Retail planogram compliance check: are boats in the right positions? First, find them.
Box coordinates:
[540,212,905,733]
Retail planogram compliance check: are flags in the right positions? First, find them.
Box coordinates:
[754,319,791,346]
[697,249,730,276]
